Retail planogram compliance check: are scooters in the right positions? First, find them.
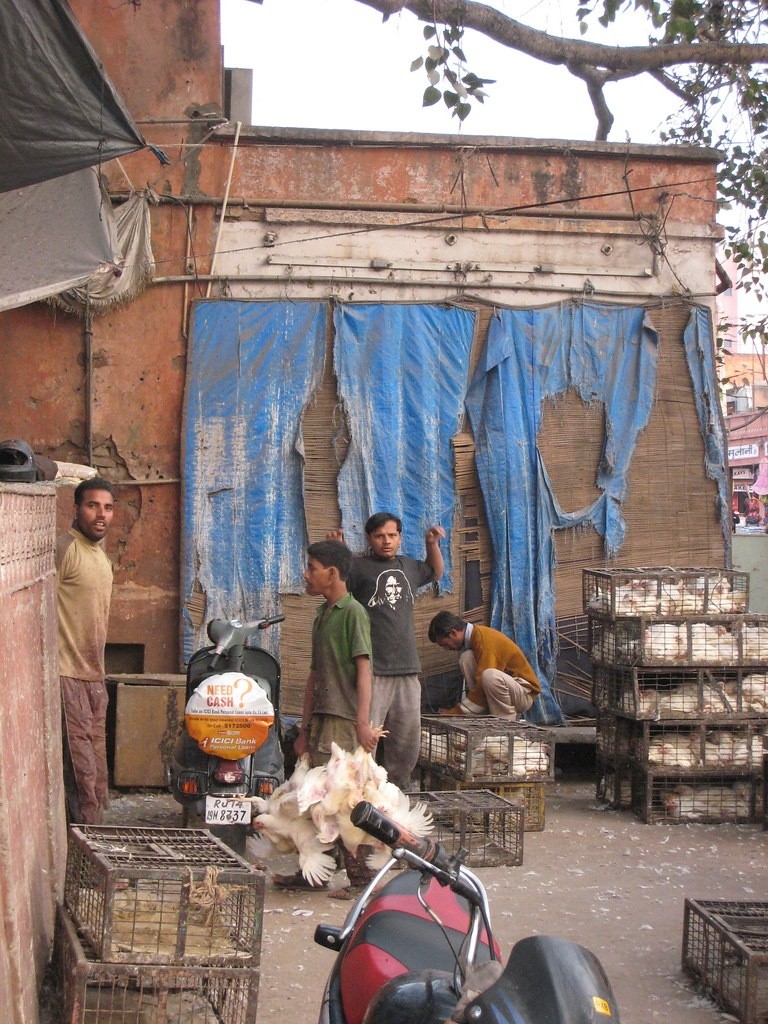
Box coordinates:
[316,802,620,1022]
[168,612,296,853]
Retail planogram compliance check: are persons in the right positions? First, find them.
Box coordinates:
[55,477,116,823]
[271,540,380,899]
[328,513,446,798]
[427,610,542,723]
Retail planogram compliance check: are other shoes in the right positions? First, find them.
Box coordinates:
[274,872,329,890]
[337,886,367,899]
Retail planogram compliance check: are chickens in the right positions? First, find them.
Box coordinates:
[589,573,768,818]
[421,717,551,777]
[227,721,434,887]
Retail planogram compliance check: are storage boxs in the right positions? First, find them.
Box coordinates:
[64,821,265,968]
[415,714,555,782]
[588,616,768,666]
[405,789,524,868]
[49,899,260,1024]
[681,897,768,1024]
[592,666,768,721]
[597,715,768,774]
[582,567,750,620]
[419,768,546,833]
[596,764,768,825]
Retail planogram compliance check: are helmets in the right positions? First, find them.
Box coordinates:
[0,439,37,483]
